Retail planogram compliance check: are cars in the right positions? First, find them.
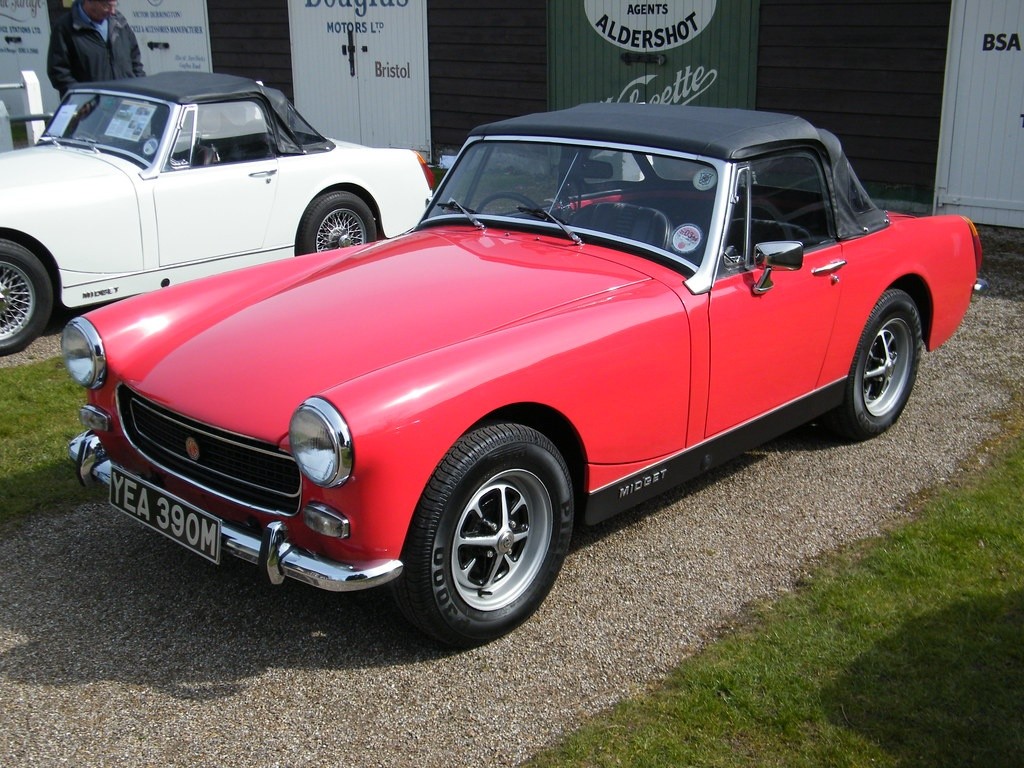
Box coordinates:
[0,65,437,354]
[56,100,985,645]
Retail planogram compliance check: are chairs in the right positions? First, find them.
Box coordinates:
[216,119,271,163]
[727,218,812,270]
[570,202,675,251]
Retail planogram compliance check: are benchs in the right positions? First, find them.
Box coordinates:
[643,180,831,246]
